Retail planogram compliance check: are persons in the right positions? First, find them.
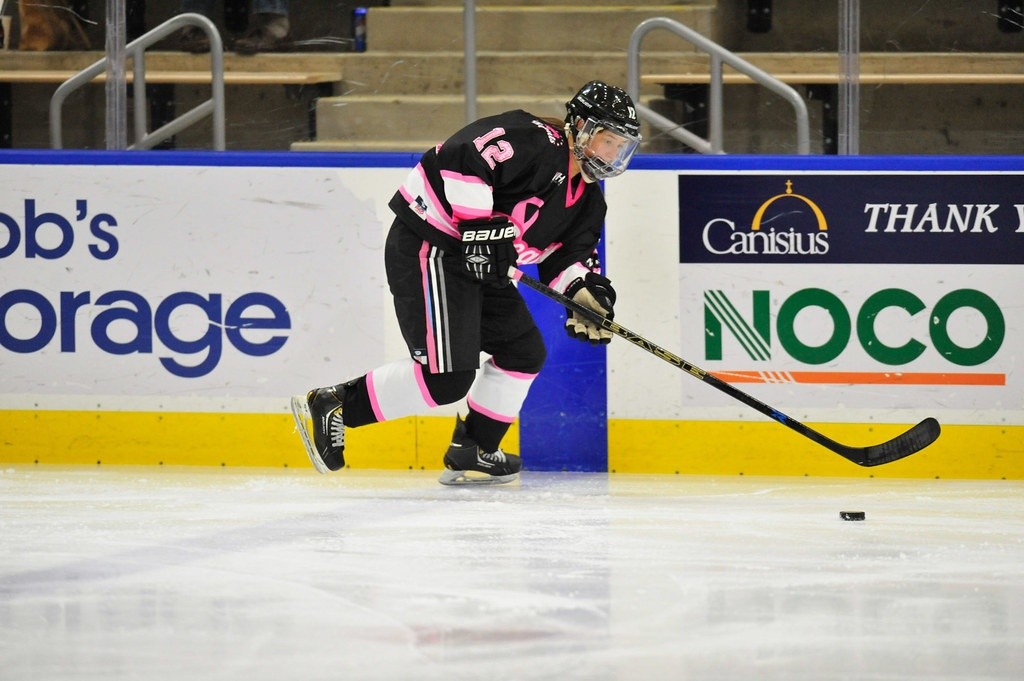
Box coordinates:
[291,80,642,486]
[169,1,291,54]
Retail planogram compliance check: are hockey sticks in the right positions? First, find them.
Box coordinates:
[507,263,942,468]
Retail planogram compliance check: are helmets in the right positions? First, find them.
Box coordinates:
[567,82,642,183]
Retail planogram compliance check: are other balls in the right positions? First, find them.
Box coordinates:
[838,510,866,522]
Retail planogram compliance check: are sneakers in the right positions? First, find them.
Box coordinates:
[441,414,525,488]
[290,381,352,475]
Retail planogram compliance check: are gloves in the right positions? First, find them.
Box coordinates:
[458,217,519,289]
[560,273,617,344]
